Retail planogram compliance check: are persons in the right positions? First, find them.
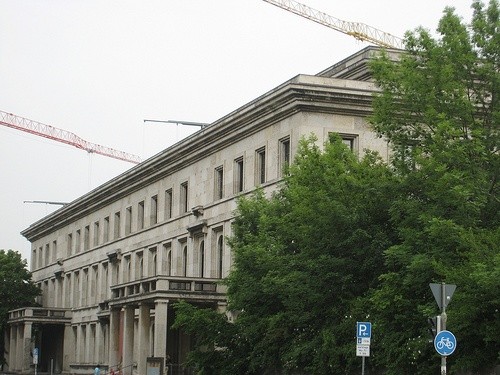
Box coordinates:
[94,364,100,375]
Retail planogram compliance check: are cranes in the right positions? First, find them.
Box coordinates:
[145,119,211,129]
[263,0,407,49]
[24,200,69,205]
[0,111,144,164]
